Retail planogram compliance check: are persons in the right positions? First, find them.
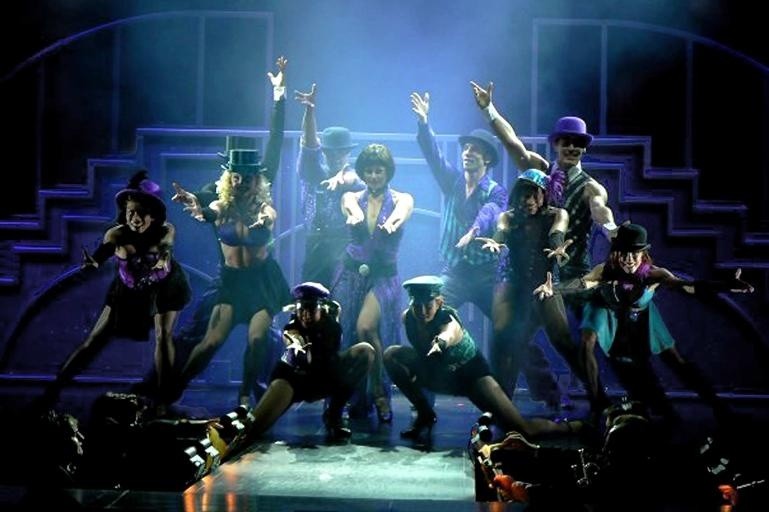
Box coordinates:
[27,167,194,407]
[131,51,294,396]
[480,393,768,512]
[380,273,604,441]
[147,277,380,442]
[134,147,295,413]
[529,223,758,418]
[407,108,575,418]
[286,85,361,291]
[475,164,613,429]
[327,144,409,427]
[470,83,642,414]
[1,389,153,511]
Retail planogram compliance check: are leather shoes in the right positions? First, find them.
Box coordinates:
[372,393,393,424]
[543,399,576,414]
[567,415,596,439]
[715,400,754,421]
[587,394,619,415]
[349,400,369,419]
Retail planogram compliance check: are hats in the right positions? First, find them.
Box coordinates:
[547,115,594,147]
[217,133,264,162]
[289,282,332,304]
[113,178,169,229]
[609,223,654,253]
[317,126,358,153]
[516,168,555,190]
[458,128,502,171]
[400,273,445,307]
[219,148,272,175]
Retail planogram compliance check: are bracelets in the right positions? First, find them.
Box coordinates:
[602,218,617,236]
[481,103,499,123]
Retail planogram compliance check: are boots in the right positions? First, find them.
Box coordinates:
[395,374,438,444]
[43,343,100,402]
[321,357,368,441]
[222,409,275,463]
